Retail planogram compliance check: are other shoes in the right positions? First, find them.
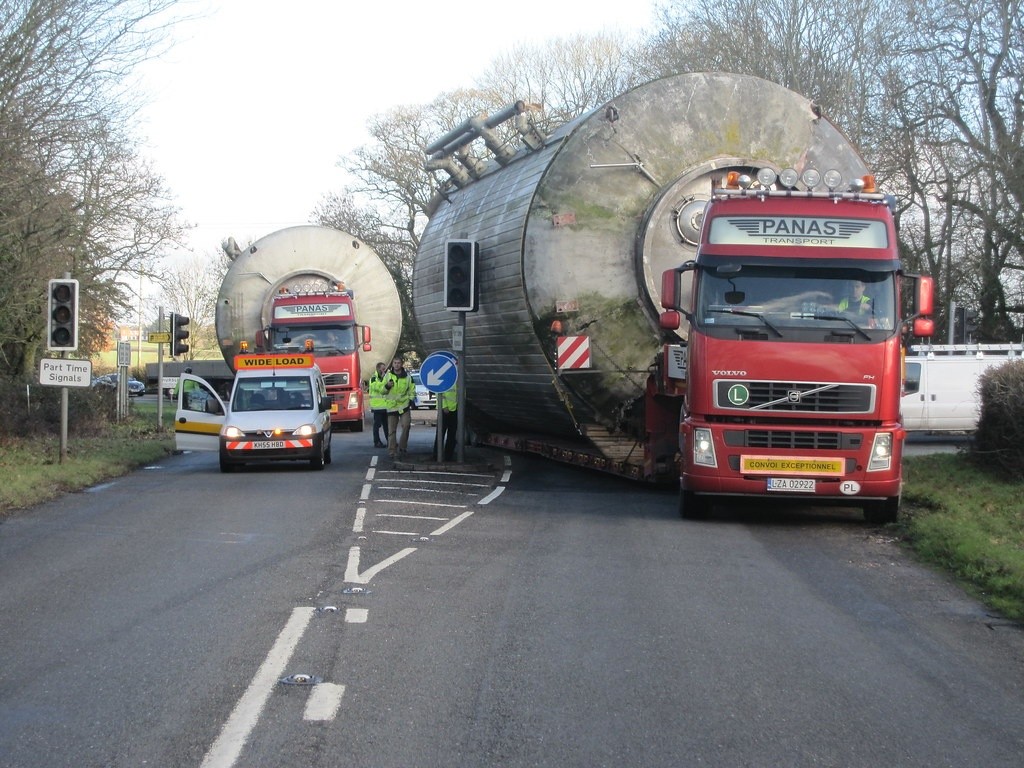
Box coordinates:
[374,441,387,448]
[388,452,396,458]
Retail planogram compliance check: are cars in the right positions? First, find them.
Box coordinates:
[411,375,438,410]
[95,372,145,396]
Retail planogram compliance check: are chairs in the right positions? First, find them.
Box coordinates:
[286,392,305,410]
[248,393,266,411]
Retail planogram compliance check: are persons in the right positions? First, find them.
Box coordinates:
[432,380,458,463]
[326,330,339,346]
[837,277,884,318]
[380,356,417,458]
[173,367,199,410]
[369,362,399,448]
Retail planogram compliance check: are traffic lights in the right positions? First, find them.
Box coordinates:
[170,312,189,358]
[48,279,79,351]
[444,239,479,312]
[959,308,978,343]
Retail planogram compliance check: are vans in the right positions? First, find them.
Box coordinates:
[901,343,1024,441]
[174,355,333,472]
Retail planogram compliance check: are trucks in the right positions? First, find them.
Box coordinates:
[215,224,403,432]
[411,68,934,526]
